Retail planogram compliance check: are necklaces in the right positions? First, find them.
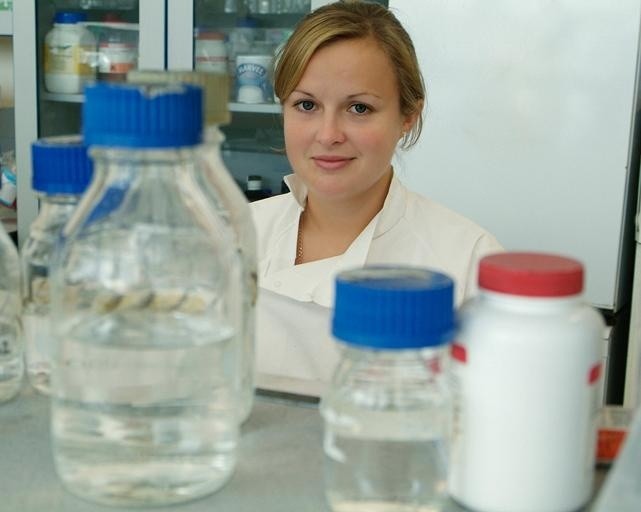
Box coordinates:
[296,213,306,266]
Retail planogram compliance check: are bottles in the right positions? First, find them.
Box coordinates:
[446,253,607,512]
[0,8,281,509]
[316,266,457,512]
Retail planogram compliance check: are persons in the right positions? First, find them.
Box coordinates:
[245,1,508,400]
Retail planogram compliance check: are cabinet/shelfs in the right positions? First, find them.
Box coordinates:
[11,0,336,260]
